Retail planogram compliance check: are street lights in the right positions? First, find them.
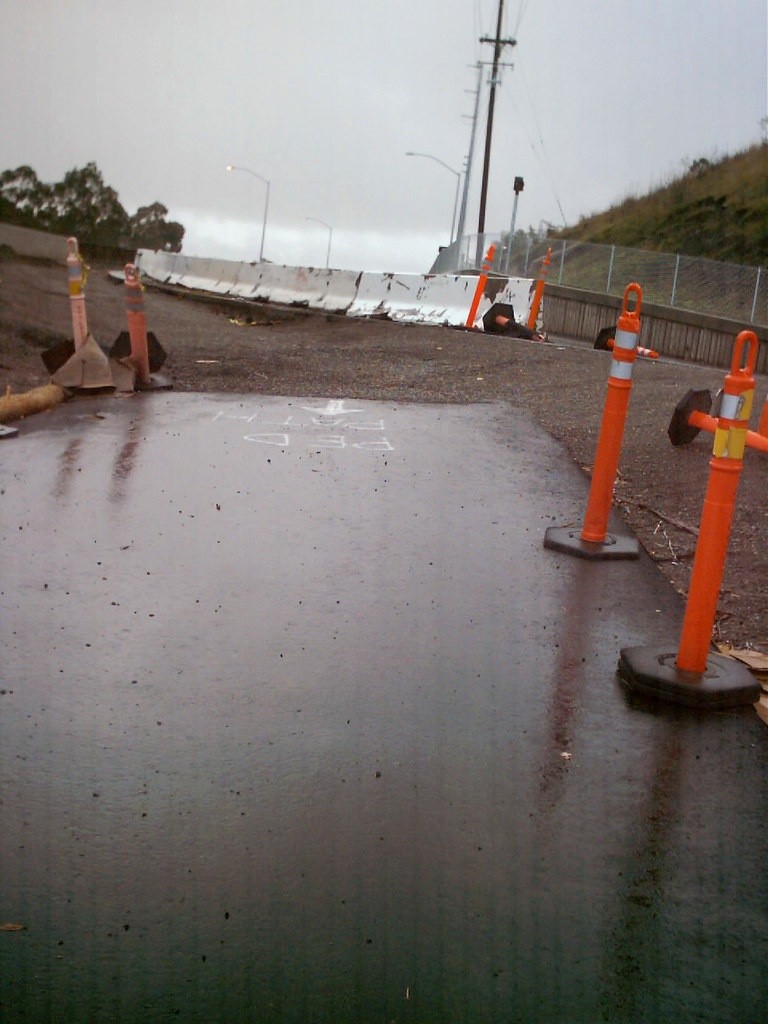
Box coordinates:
[405,152,462,247]
[225,165,271,263]
[304,216,333,268]
